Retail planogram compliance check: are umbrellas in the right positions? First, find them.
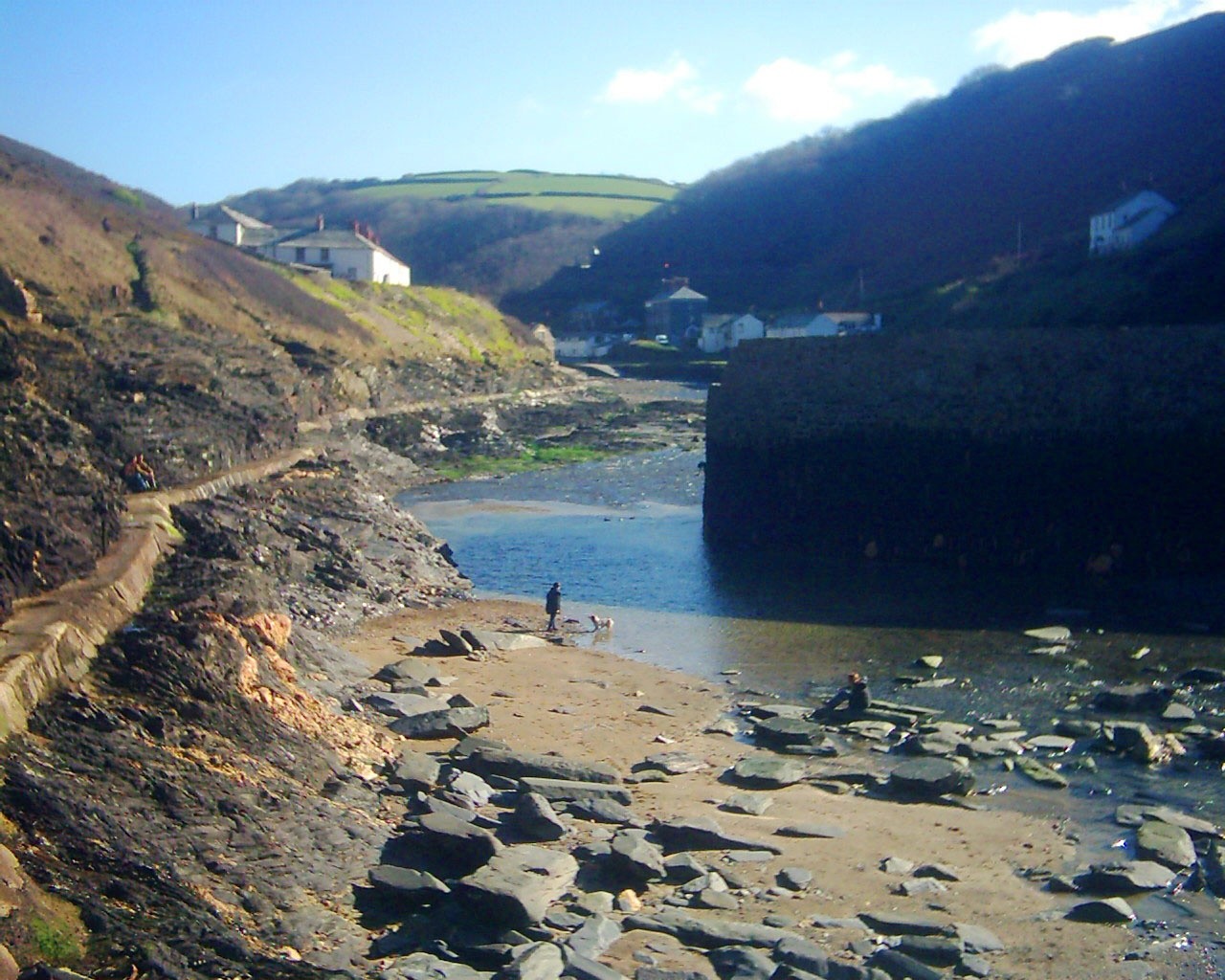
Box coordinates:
[669,286,706,298]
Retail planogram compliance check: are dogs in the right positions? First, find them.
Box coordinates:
[588,615,614,633]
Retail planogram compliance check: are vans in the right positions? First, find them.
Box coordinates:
[655,334,669,345]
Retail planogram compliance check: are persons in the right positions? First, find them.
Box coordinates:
[820,672,871,710]
[122,453,157,491]
[546,582,561,631]
